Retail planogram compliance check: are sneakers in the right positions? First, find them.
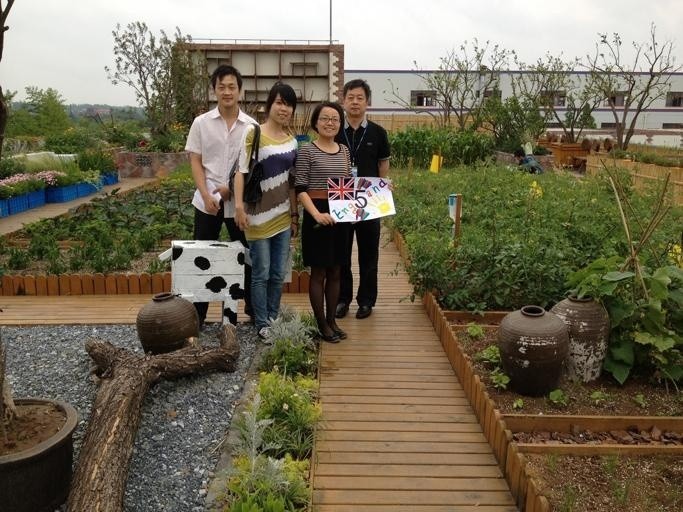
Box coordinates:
[257,327,274,344]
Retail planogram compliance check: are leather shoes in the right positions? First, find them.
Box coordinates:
[356,305,372,319]
[318,325,347,343]
[335,303,349,319]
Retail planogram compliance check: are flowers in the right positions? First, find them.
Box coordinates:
[0,169,79,200]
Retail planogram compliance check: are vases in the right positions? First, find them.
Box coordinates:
[494,292,611,399]
[0,184,78,218]
[136,292,200,356]
[1,394,79,512]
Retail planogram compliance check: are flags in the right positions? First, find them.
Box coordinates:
[327,177,355,201]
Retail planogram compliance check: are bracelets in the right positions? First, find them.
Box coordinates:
[292,221,300,226]
[290,213,300,219]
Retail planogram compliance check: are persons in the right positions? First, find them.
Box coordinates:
[183,62,256,328]
[232,80,301,345]
[330,78,393,320]
[296,98,395,344]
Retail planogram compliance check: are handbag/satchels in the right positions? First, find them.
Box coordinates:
[229,123,263,203]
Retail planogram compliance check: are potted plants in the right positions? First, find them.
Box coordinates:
[73,144,120,199]
